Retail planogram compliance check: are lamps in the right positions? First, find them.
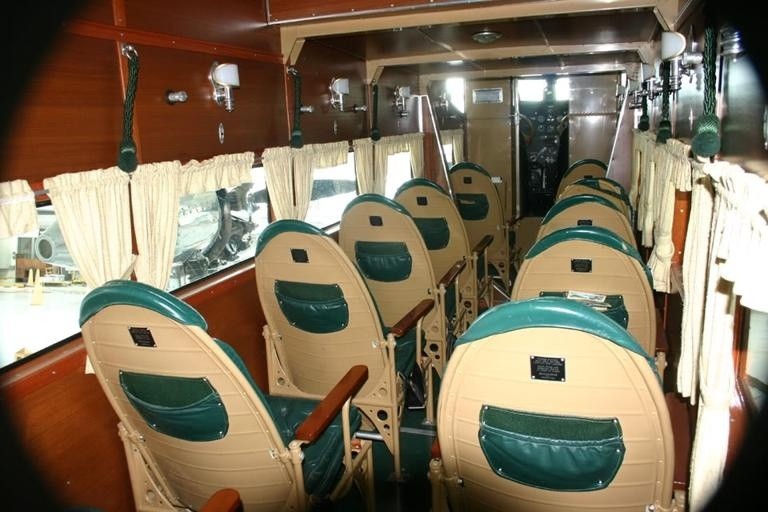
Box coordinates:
[394,85,411,117]
[615,30,704,110]
[207,60,241,113]
[328,77,350,113]
